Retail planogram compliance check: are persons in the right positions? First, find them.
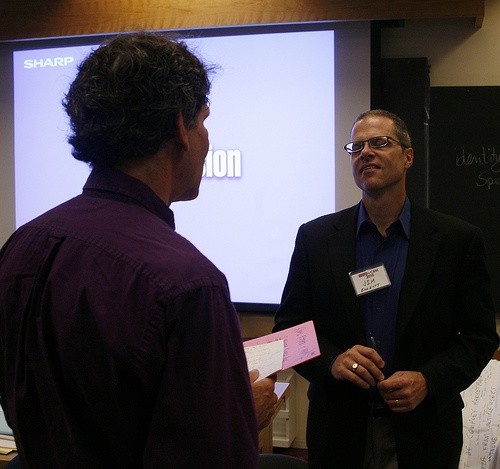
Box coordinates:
[271,108,498,469]
[0,30,279,469]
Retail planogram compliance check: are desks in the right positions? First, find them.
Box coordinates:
[258,382,291,455]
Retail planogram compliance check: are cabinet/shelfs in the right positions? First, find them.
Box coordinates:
[273,373,296,448]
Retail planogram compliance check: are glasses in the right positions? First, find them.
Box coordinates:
[344,136,408,156]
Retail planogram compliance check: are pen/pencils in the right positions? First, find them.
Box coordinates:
[369,332,389,381]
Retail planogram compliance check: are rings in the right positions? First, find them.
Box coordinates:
[395,399,399,408]
[352,363,360,374]
